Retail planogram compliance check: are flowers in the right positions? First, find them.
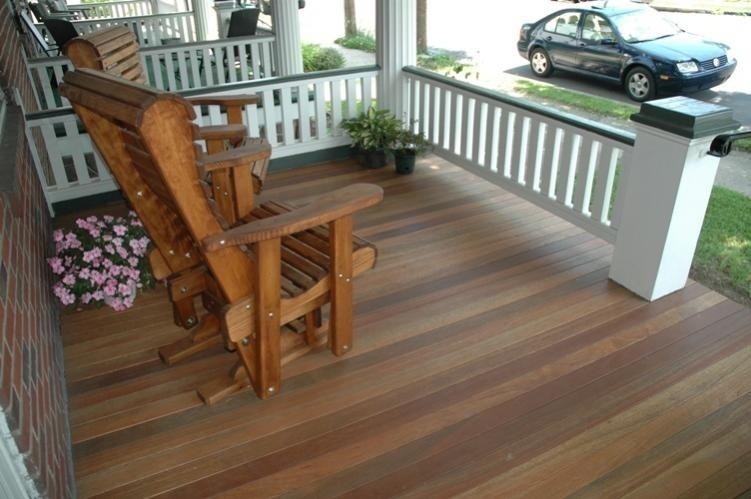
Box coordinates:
[46,210,150,313]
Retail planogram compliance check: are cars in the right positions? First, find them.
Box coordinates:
[517,2,738,100]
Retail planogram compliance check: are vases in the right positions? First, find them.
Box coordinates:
[102,284,136,308]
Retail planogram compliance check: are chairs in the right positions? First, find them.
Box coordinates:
[58,66,385,408]
[20,0,260,88]
[66,26,272,327]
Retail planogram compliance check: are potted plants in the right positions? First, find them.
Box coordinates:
[338,107,426,174]
